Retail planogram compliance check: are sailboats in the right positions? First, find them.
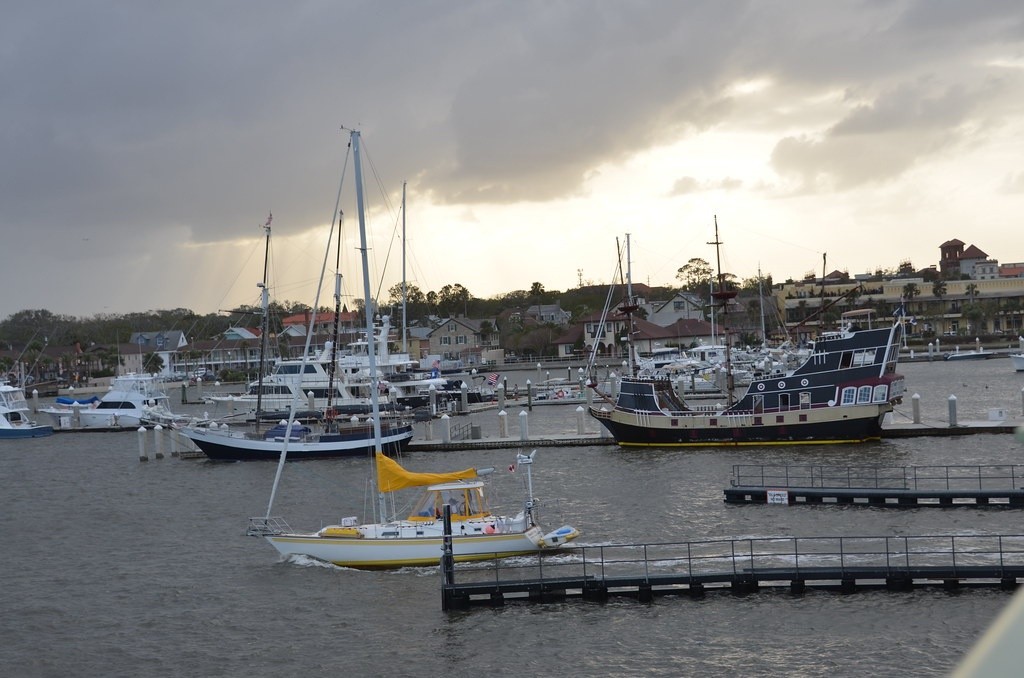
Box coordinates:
[249,124,586,567]
[636,269,813,375]
[587,215,909,441]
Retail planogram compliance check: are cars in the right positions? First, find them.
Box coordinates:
[195,368,211,376]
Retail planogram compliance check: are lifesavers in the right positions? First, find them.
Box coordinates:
[325,408,337,420]
[557,390,564,398]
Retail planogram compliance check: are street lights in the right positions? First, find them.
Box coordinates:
[227,351,231,371]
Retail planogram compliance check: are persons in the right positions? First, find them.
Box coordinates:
[431,360,440,378]
[449,498,459,514]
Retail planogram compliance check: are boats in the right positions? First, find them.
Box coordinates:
[0,380,54,439]
[637,360,748,393]
[38,372,189,430]
[947,351,996,360]
[111,208,485,461]
[1008,353,1024,371]
[24,380,57,398]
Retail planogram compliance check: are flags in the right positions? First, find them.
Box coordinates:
[488,373,500,386]
